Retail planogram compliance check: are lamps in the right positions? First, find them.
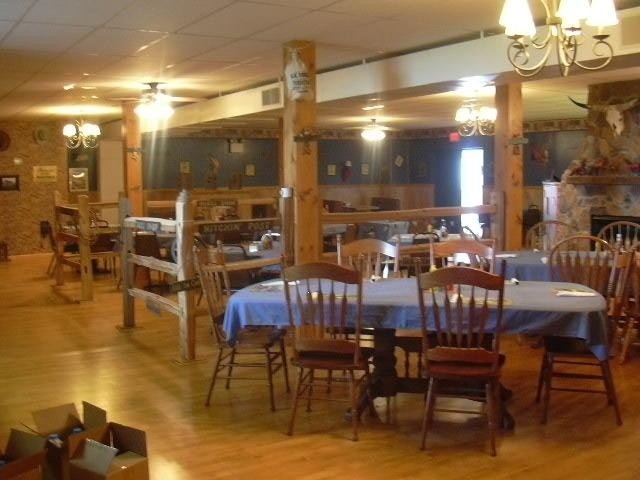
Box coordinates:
[498,0,620,77]
[455,107,496,137]
[134,81,173,119]
[361,119,385,141]
[63,117,101,151]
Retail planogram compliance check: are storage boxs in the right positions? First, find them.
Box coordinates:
[0,401,150,480]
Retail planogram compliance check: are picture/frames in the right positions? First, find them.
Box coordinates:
[69,168,89,192]
[0,175,19,191]
